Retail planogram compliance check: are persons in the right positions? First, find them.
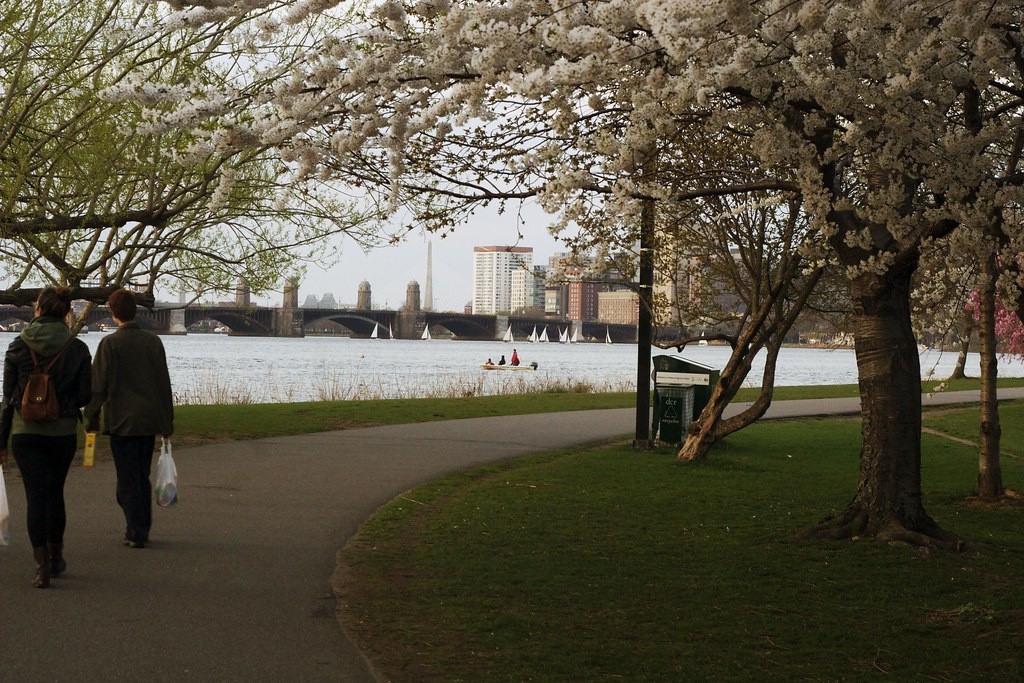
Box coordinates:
[83,289,175,550]
[497,355,506,365]
[0,284,93,586]
[486,358,494,365]
[510,348,520,366]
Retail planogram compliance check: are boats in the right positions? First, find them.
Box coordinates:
[479,364,536,371]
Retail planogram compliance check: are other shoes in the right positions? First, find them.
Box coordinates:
[123,537,144,548]
[32,567,49,588]
[48,553,65,576]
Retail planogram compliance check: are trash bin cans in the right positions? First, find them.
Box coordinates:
[653,354,721,443]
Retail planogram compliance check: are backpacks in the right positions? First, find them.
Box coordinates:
[19,337,74,422]
